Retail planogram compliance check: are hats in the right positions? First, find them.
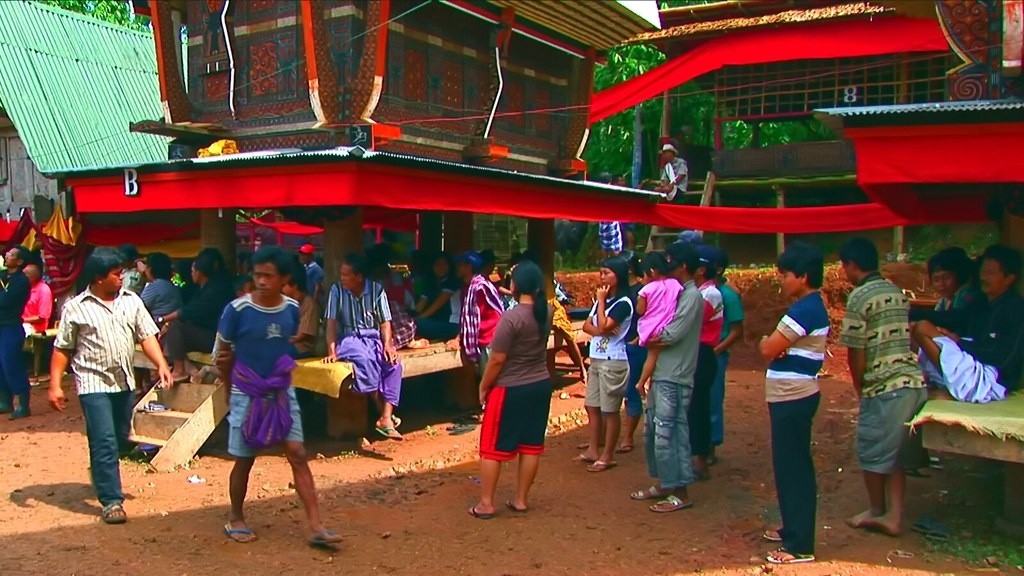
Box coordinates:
[658,144,678,156]
[454,250,483,267]
[296,244,316,252]
[692,245,716,260]
[673,230,703,247]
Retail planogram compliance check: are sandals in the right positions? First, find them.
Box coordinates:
[102,502,127,522]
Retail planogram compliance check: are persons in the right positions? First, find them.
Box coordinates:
[49,247,174,525]
[0,245,31,419]
[521,251,587,383]
[617,242,747,484]
[324,252,403,440]
[837,236,928,538]
[22,264,54,386]
[457,249,506,422]
[759,240,830,564]
[906,241,1024,405]
[571,256,633,472]
[630,244,704,513]
[466,262,554,520]
[596,130,688,205]
[119,241,530,367]
[212,245,346,545]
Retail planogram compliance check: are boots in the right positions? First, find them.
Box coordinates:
[9,394,31,419]
[0,390,12,412]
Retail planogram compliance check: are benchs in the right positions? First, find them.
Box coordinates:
[905,387,1024,540]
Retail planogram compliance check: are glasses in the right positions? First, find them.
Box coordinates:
[6,252,17,257]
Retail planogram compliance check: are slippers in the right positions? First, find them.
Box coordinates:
[631,486,669,499]
[375,414,402,439]
[650,495,693,512]
[572,453,598,462]
[912,514,949,538]
[615,444,633,452]
[307,529,343,544]
[693,470,710,480]
[447,424,475,434]
[224,524,256,542]
[766,547,815,563]
[587,460,617,472]
[907,467,930,477]
[763,529,783,541]
[507,500,527,512]
[468,506,492,519]
